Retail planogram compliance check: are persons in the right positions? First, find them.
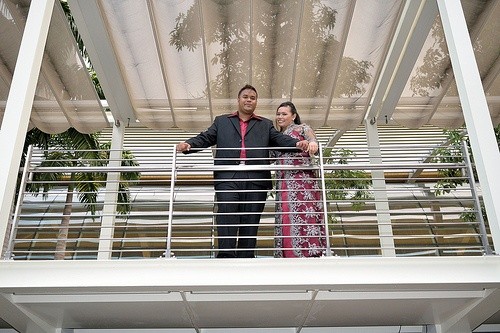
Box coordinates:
[269,102,328,258]
[177,85,309,258]
